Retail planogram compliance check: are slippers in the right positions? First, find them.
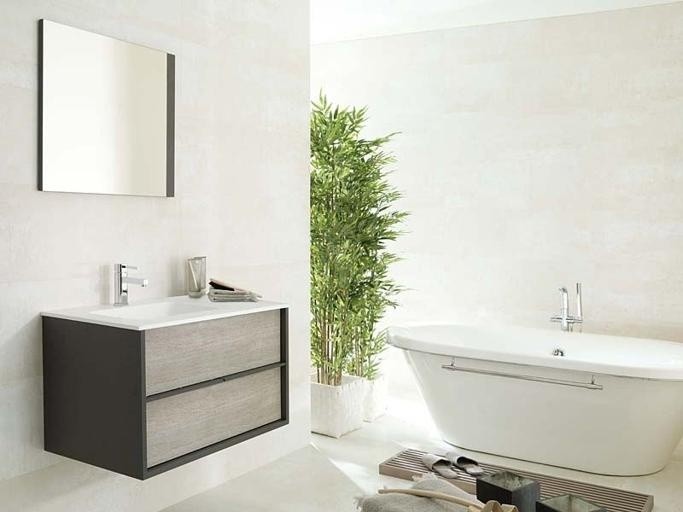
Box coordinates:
[445,450,485,476]
[420,452,458,479]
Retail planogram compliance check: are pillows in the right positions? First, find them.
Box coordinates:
[355,473,492,512]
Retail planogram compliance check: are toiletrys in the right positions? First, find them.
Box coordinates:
[187,259,202,298]
[195,256,207,293]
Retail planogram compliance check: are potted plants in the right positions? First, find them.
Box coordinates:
[310,88,408,439]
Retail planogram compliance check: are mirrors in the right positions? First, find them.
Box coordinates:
[38,20,175,197]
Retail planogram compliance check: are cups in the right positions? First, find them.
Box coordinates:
[187,256,207,299]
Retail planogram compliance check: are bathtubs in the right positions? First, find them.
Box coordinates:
[382,323,683,478]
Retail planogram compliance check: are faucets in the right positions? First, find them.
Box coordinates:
[558,286,569,331]
[115,262,147,305]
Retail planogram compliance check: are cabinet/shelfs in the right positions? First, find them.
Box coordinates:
[38,293,288,480]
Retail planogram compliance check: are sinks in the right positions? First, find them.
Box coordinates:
[89,300,217,321]
